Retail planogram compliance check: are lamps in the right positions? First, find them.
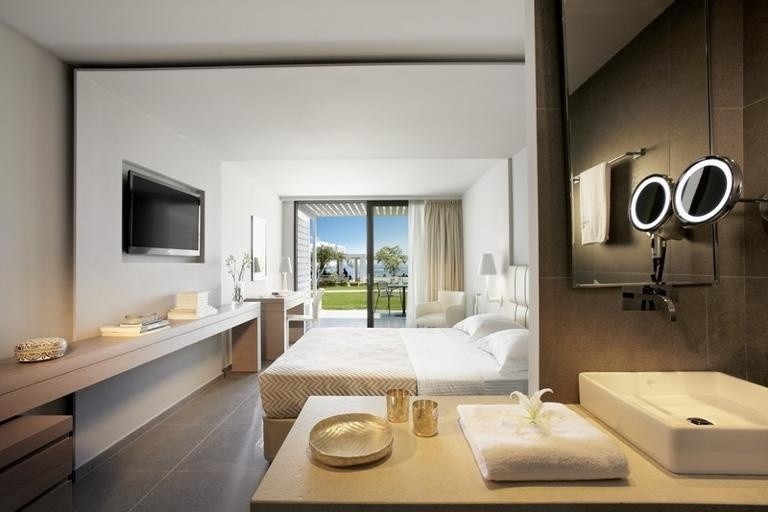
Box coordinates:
[277,255,293,293]
[478,254,504,306]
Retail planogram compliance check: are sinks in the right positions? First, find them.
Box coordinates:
[575,368,768,478]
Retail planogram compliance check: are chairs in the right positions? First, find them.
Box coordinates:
[414,290,466,329]
[288,287,325,332]
[373,282,403,316]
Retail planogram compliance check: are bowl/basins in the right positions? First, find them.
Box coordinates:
[308,411,394,466]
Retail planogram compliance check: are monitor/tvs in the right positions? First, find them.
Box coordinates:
[127,169,201,257]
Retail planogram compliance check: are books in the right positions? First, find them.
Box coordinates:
[100,312,172,338]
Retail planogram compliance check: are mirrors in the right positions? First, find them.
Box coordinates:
[628,174,689,239]
[560,1,719,292]
[671,154,767,231]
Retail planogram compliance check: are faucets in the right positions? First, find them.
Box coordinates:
[655,292,678,323]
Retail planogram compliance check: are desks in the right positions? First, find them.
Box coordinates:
[250,291,305,362]
[389,284,410,317]
[1,302,263,512]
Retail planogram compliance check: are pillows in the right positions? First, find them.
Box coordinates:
[476,328,528,376]
[452,313,527,341]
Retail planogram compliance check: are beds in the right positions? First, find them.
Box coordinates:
[258,265,529,462]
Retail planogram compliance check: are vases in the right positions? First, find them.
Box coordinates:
[232,282,246,305]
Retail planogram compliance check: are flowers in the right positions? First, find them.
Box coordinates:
[224,254,258,300]
[506,386,559,435]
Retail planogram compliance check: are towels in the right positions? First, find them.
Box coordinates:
[454,401,631,485]
[580,163,611,244]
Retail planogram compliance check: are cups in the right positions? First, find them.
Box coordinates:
[386,388,409,424]
[410,399,439,437]
